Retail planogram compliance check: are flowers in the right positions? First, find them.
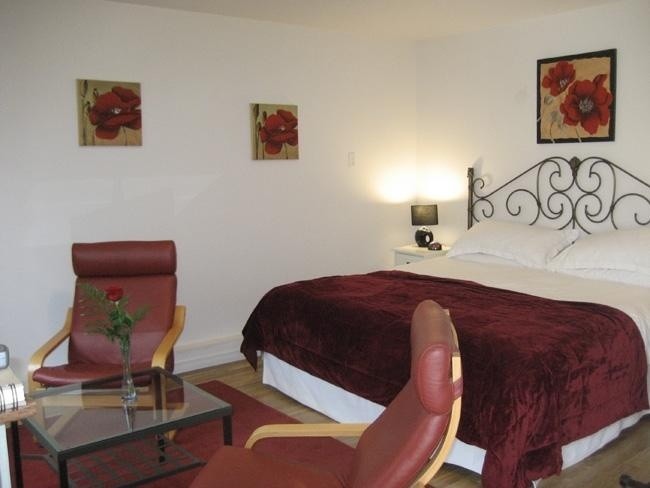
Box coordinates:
[75,282,158,349]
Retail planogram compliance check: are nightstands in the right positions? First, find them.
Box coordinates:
[395,244,450,265]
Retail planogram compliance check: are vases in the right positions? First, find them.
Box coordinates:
[121,402,136,430]
[120,343,137,400]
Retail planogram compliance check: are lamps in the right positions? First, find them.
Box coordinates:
[411,205,438,247]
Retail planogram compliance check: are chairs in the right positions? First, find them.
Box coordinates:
[189,299,464,488]
[28,241,185,444]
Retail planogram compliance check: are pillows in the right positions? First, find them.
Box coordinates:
[447,220,580,268]
[546,229,650,285]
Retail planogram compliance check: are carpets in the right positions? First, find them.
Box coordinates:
[6,381,434,488]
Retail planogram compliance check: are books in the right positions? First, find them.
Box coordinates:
[0,362,26,411]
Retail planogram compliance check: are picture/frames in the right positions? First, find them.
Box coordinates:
[536,49,617,143]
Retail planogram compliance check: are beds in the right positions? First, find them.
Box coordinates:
[261,157,650,488]
